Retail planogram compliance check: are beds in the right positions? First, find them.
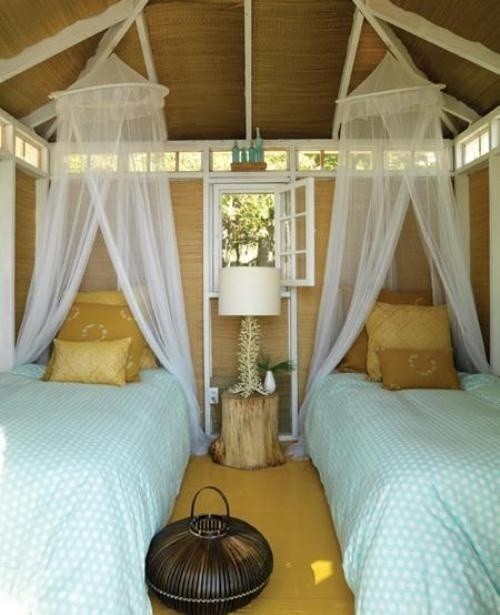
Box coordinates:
[0,361,191,615]
[305,367,499,615]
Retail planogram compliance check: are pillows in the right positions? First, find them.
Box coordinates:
[41,290,160,387]
[336,283,464,393]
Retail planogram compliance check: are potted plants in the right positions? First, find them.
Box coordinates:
[256,354,297,394]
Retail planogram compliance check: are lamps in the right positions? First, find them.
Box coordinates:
[217,266,282,398]
